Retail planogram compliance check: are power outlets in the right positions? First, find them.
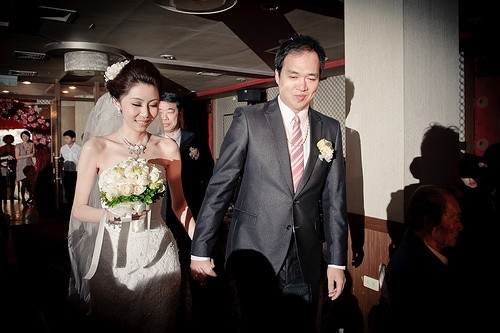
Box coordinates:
[363,275,380,292]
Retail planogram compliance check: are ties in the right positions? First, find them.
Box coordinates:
[288,115,305,195]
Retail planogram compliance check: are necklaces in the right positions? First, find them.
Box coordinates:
[121,133,149,158]
[284,116,310,147]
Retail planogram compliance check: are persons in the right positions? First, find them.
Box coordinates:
[58,59,215,333]
[0,131,51,208]
[367,143,500,333]
[190,34,348,301]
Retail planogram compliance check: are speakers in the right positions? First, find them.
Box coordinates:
[237,89,266,103]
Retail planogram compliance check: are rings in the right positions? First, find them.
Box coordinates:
[194,274,198,277]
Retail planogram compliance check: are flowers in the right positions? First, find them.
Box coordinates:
[316,138,334,162]
[0,98,52,149]
[188,147,200,161]
[98,157,167,233]
